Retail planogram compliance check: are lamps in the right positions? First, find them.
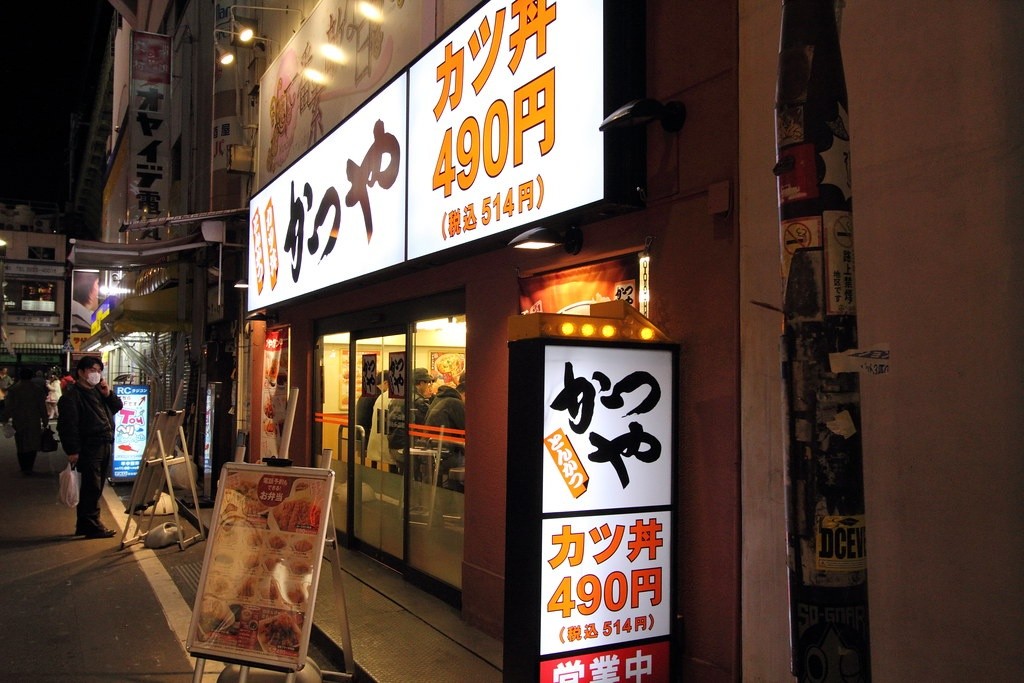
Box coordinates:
[246,312,279,323]
[230,5,305,41]
[213,28,282,65]
[599,96,687,134]
[508,226,582,255]
[234,280,248,288]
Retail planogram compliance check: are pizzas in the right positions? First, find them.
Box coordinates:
[436,353,465,376]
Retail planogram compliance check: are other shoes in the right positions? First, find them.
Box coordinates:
[75,528,86,536]
[90,528,116,538]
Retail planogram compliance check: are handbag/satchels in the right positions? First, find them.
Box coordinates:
[41,425,57,452]
[58,462,81,508]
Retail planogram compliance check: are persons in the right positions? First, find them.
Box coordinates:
[46,373,62,420]
[0,367,14,428]
[33,364,77,394]
[55,355,123,538]
[0,367,49,479]
[355,365,468,493]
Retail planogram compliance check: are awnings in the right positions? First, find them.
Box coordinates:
[100,283,196,351]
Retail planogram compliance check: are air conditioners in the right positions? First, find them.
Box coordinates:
[4,222,21,230]
[33,219,50,233]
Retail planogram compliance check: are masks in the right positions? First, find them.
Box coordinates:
[81,372,100,385]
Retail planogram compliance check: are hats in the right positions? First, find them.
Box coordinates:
[412,367,436,382]
